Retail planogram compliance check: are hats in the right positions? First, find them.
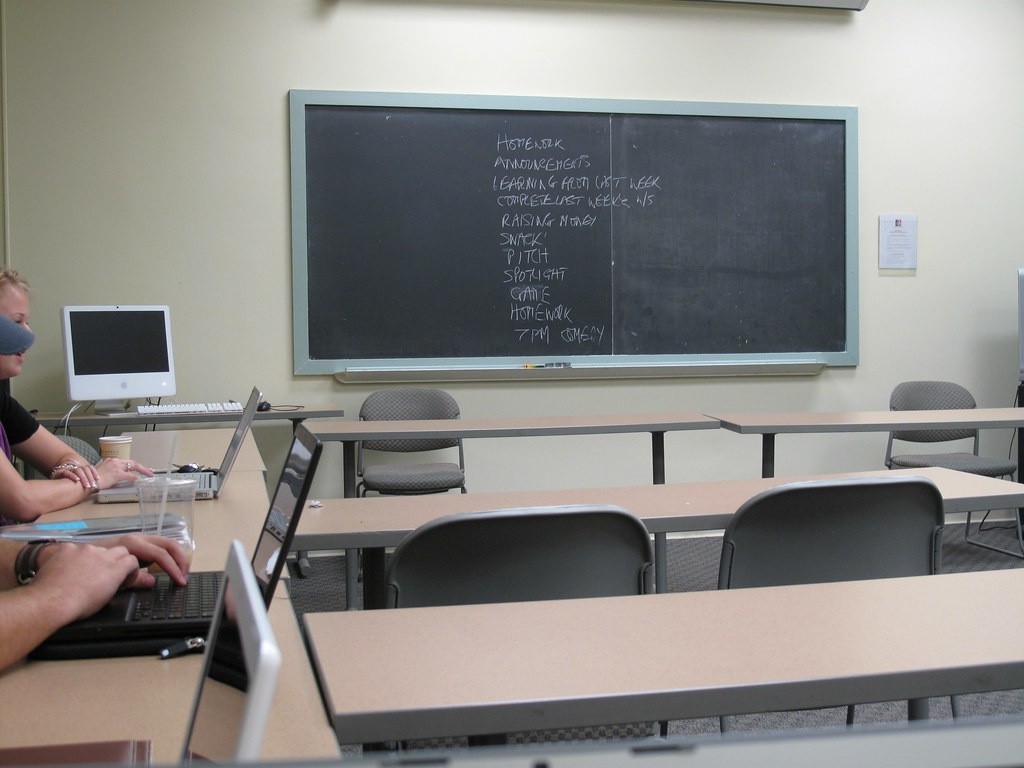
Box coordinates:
[0,314,35,354]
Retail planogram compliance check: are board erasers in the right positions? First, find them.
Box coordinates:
[544,362,572,368]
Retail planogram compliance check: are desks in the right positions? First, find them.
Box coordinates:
[702,407,1024,477]
[304,566,1024,759]
[301,415,724,612]
[32,404,344,578]
[291,465,1024,612]
[0,469,342,768]
[102,424,268,481]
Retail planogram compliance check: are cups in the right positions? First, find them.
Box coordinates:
[134,476,199,572]
[98,435,132,461]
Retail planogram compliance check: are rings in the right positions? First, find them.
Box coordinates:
[70,467,77,471]
[126,463,131,470]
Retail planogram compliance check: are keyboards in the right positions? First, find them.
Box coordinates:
[137,402,244,415]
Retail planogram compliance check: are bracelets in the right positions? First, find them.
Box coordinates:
[15,538,61,585]
[50,463,73,478]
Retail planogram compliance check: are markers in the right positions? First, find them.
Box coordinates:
[523,364,544,368]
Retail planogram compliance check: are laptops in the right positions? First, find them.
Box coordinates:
[42,422,324,644]
[179,538,284,768]
[99,386,264,502]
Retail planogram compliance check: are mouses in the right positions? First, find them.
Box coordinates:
[256,401,271,411]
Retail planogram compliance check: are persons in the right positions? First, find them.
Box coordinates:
[0,263,154,524]
[0,533,192,679]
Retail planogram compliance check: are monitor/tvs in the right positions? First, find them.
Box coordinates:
[60,305,176,415]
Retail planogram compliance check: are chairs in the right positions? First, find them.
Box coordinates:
[366,505,667,757]
[355,391,466,505]
[716,482,959,736]
[883,382,1020,543]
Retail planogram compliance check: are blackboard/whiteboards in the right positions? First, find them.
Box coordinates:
[288,89,859,385]
